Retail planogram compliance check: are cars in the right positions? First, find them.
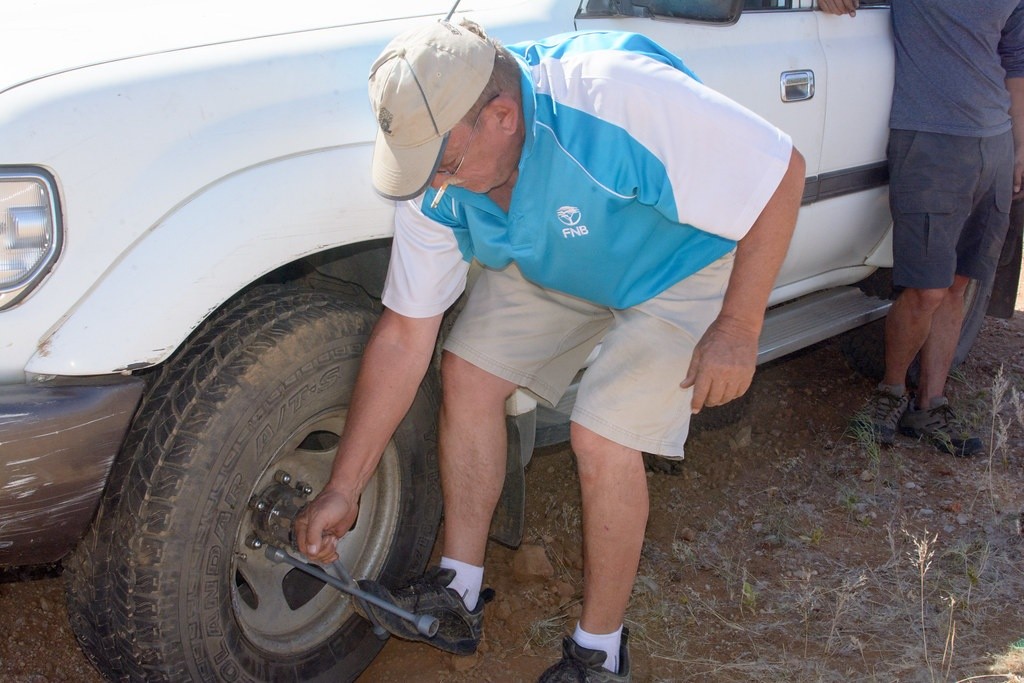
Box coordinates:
[0,1,1024,683]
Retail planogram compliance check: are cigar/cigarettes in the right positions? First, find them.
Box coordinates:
[430,181,451,210]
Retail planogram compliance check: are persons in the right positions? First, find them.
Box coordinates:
[821,0,1024,455]
[296,19,806,683]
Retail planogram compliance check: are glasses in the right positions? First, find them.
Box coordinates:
[437,94,500,176]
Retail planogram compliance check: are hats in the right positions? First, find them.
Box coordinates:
[367,18,496,202]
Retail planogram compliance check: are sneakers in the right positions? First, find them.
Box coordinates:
[536,626,631,683]
[851,380,909,443]
[355,566,495,655]
[898,391,983,455]
